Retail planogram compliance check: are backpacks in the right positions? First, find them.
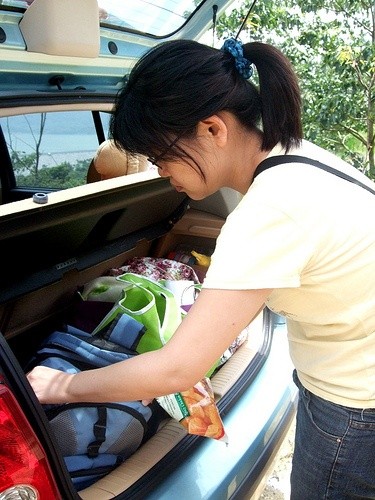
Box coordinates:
[25,311,156,489]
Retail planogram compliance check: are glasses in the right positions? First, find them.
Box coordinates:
[147,136,179,170]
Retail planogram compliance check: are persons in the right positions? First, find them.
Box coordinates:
[26,38,374,500]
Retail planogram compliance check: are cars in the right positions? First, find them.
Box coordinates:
[0,0,298,500]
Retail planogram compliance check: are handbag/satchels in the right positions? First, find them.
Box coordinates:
[87,273,176,354]
